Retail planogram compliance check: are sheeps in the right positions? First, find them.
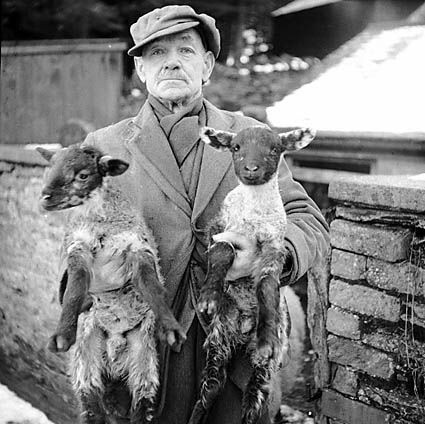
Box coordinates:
[34,141,188,424]
[189,124,319,424]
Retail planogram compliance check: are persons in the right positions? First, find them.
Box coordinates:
[57,4,332,424]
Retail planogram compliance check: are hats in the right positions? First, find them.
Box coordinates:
[127,5,221,59]
